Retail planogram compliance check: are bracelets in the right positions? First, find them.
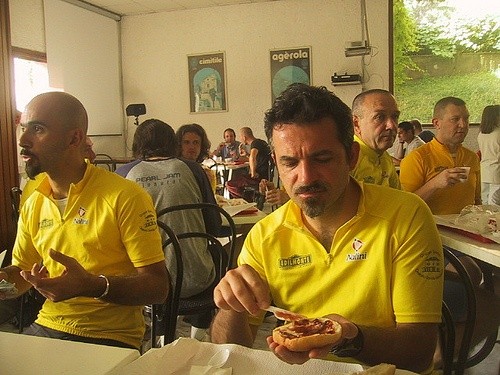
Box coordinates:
[399,142,405,143]
[93,274,110,299]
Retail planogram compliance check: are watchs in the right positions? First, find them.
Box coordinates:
[328,323,363,357]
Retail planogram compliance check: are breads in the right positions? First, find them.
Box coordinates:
[272,317,341,352]
[350,363,396,375]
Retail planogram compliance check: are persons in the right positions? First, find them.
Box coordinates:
[210,82,443,375]
[0,92,291,350]
[349,88,500,215]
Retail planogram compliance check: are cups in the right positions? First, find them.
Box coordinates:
[454,166,470,183]
[225,157,233,163]
[216,156,222,164]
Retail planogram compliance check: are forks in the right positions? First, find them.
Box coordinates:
[260,304,308,322]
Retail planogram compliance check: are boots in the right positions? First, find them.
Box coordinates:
[160,336,164,347]
[190,326,206,342]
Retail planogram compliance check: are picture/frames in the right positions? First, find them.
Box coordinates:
[186,50,229,114]
[267,44,313,109]
[389,0,500,128]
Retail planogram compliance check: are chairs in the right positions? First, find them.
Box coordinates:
[93,150,500,375]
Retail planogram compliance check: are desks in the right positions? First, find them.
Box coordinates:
[204,158,250,200]
[95,155,136,172]
[432,214,500,270]
[0,331,139,375]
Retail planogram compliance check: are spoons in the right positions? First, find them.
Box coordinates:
[202,348,230,375]
